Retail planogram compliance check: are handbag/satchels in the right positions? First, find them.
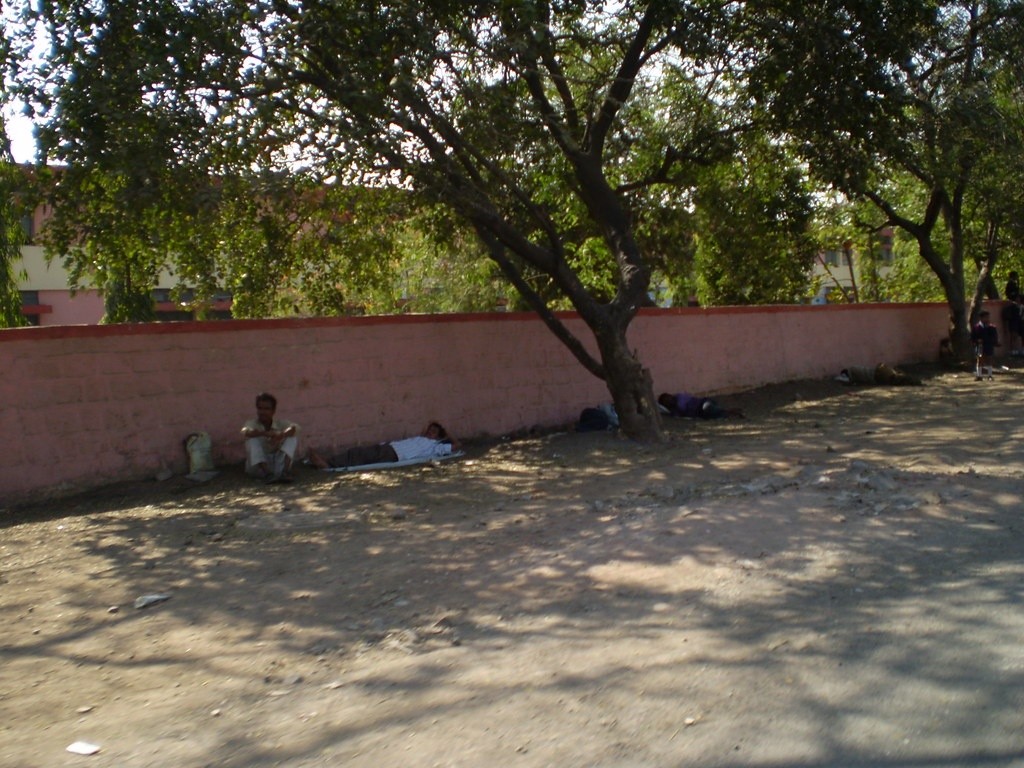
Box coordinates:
[186,430,215,474]
[977,342,984,367]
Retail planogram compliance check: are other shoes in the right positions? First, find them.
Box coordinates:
[281,469,294,482]
[988,376,994,382]
[264,472,279,484]
[975,376,983,381]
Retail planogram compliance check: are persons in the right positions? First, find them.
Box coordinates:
[940,339,958,363]
[841,367,922,386]
[568,402,620,437]
[1002,271,1024,356]
[972,311,998,380]
[240,394,301,483]
[658,393,745,419]
[310,423,459,469]
